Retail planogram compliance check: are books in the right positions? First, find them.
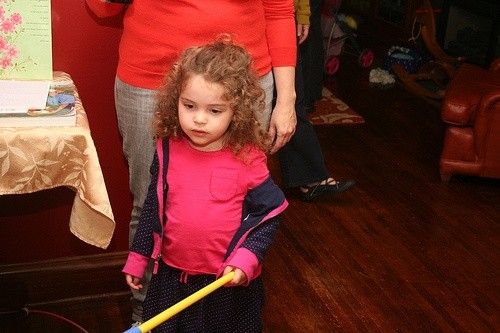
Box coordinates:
[0,80,76,128]
[0,0,53,81]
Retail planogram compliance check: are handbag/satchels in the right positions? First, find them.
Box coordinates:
[384,41,422,75]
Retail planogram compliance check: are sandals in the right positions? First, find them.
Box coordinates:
[299,177,355,201]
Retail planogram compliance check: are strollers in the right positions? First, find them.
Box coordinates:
[322,0,375,75]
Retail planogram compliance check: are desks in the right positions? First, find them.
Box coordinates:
[0,70,116,309]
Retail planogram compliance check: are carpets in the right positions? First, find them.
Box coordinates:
[307,86,366,125]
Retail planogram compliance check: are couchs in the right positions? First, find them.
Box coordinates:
[439,58,500,182]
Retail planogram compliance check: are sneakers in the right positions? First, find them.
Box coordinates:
[131,297,143,329]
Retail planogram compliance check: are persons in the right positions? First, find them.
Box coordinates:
[114,1,297,333]
[278,0,358,203]
[121,32,289,333]
